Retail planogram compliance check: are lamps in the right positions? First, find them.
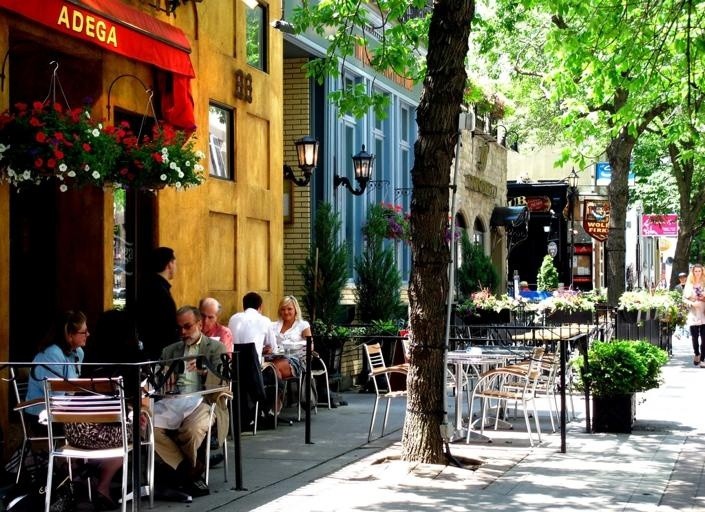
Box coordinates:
[332,143,376,196]
[285,135,322,187]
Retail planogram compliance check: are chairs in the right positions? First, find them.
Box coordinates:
[43,379,155,511]
[236,342,279,433]
[296,342,335,418]
[364,305,625,447]
[10,368,78,485]
[164,353,239,483]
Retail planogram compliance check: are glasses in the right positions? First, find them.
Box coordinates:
[74,328,89,334]
[175,319,200,331]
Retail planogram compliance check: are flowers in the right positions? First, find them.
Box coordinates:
[1,101,122,193]
[98,117,203,198]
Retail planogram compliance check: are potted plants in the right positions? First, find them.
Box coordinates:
[573,337,669,435]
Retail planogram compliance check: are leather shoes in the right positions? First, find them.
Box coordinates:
[92,488,118,511]
[694,360,699,365]
[190,480,209,492]
[210,453,223,468]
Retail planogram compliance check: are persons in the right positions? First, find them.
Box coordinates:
[136,245,178,360]
[682,261,705,370]
[673,272,690,295]
[138,304,234,506]
[25,309,86,469]
[519,280,532,293]
[227,290,280,422]
[63,309,149,510]
[269,295,313,418]
[199,296,236,366]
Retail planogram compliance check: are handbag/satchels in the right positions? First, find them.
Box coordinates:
[300,373,318,411]
[63,421,132,449]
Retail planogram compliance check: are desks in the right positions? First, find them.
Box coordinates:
[151,383,230,401]
[264,348,304,359]
